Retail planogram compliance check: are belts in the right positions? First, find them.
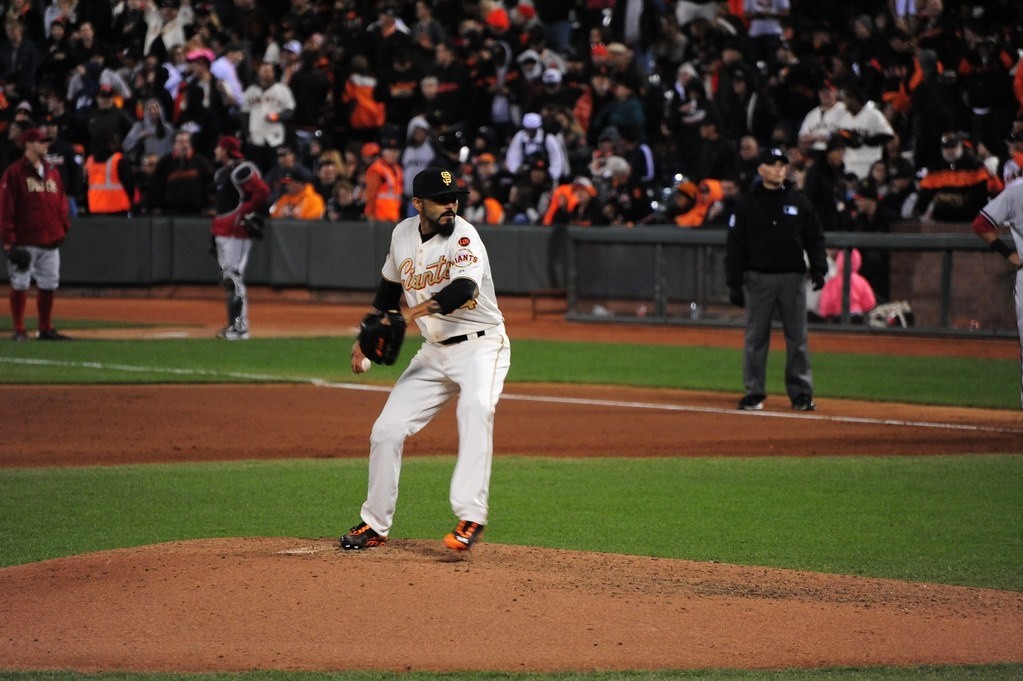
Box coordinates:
[439,330,485,345]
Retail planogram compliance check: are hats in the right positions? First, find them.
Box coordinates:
[186,46,215,63]
[413,168,470,198]
[219,136,244,158]
[542,68,562,84]
[20,128,53,143]
[756,147,788,165]
[283,40,302,57]
[940,130,960,147]
[523,112,543,129]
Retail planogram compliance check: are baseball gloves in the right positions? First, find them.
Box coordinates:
[357,305,407,366]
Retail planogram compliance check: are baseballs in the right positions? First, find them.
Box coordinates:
[361,356,372,372]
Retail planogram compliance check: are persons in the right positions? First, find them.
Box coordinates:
[971,182,1022,409]
[0,0,1023,318]
[341,170,511,552]
[725,149,828,411]
[1,130,70,338]
[212,138,270,338]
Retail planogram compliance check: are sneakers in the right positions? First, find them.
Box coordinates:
[340,522,388,549]
[442,520,485,552]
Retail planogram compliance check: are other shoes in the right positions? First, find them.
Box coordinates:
[736,395,764,411]
[216,325,250,340]
[35,329,72,341]
[12,329,28,342]
[793,398,815,410]
